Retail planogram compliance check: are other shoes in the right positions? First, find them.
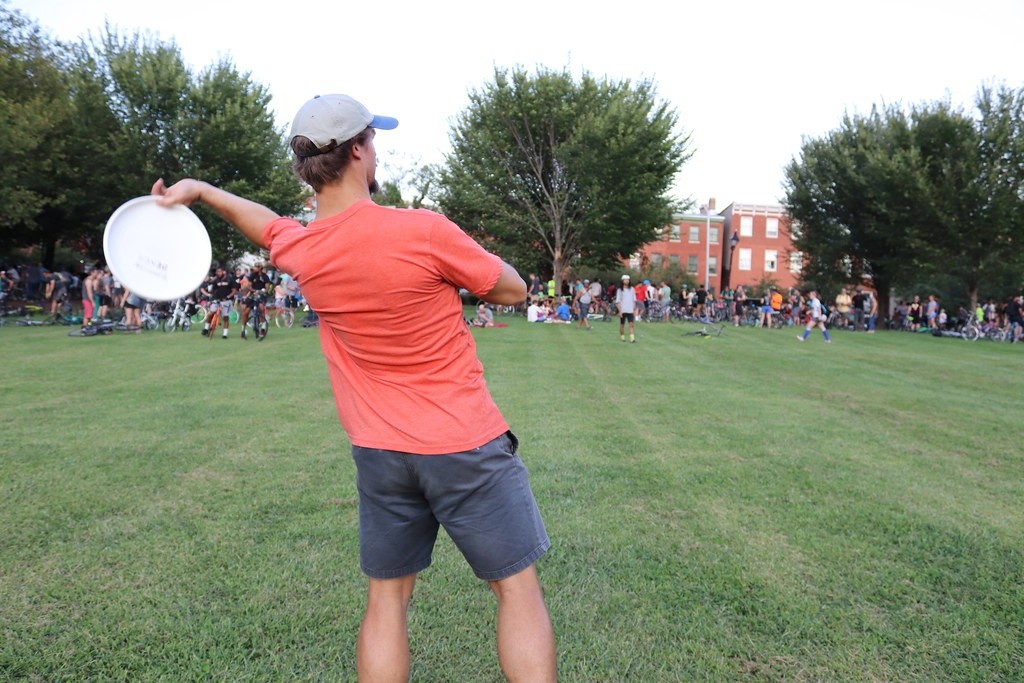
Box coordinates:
[587,326,593,329]
[629,334,635,343]
[621,335,625,342]
[223,336,227,339]
[202,329,208,335]
[241,332,245,338]
[259,329,265,337]
[796,335,804,342]
[824,340,830,343]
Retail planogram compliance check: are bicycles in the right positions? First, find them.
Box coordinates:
[265,298,294,329]
[889,316,912,333]
[68,292,239,337]
[206,296,228,341]
[960,306,1007,344]
[475,294,783,330]
[0,281,72,328]
[243,290,268,343]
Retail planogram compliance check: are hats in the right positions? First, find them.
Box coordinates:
[643,280,650,284]
[583,280,590,284]
[681,284,687,288]
[621,275,630,280]
[289,94,398,157]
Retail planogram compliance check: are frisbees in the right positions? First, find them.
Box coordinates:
[102,195,214,301]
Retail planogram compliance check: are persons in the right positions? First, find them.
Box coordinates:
[634,280,735,324]
[476,304,494,326]
[154,262,308,339]
[954,295,1024,338]
[0,262,143,334]
[797,291,831,343]
[734,284,838,329]
[827,287,878,334]
[893,293,947,331]
[151,95,557,683]
[615,274,637,342]
[528,273,618,330]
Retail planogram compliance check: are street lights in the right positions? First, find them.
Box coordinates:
[727,229,739,288]
[699,197,715,291]
